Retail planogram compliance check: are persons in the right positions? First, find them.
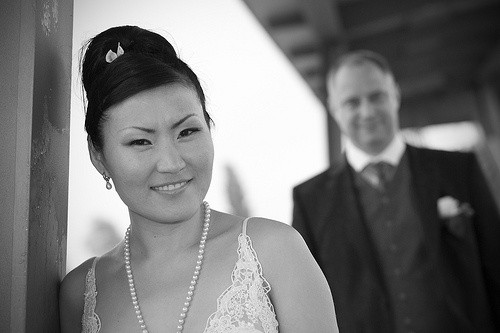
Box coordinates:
[291,48,500,333]
[56,25,340,333]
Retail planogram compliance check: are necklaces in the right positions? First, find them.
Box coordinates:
[123,201,212,333]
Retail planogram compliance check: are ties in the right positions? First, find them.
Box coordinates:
[369,163,391,192]
